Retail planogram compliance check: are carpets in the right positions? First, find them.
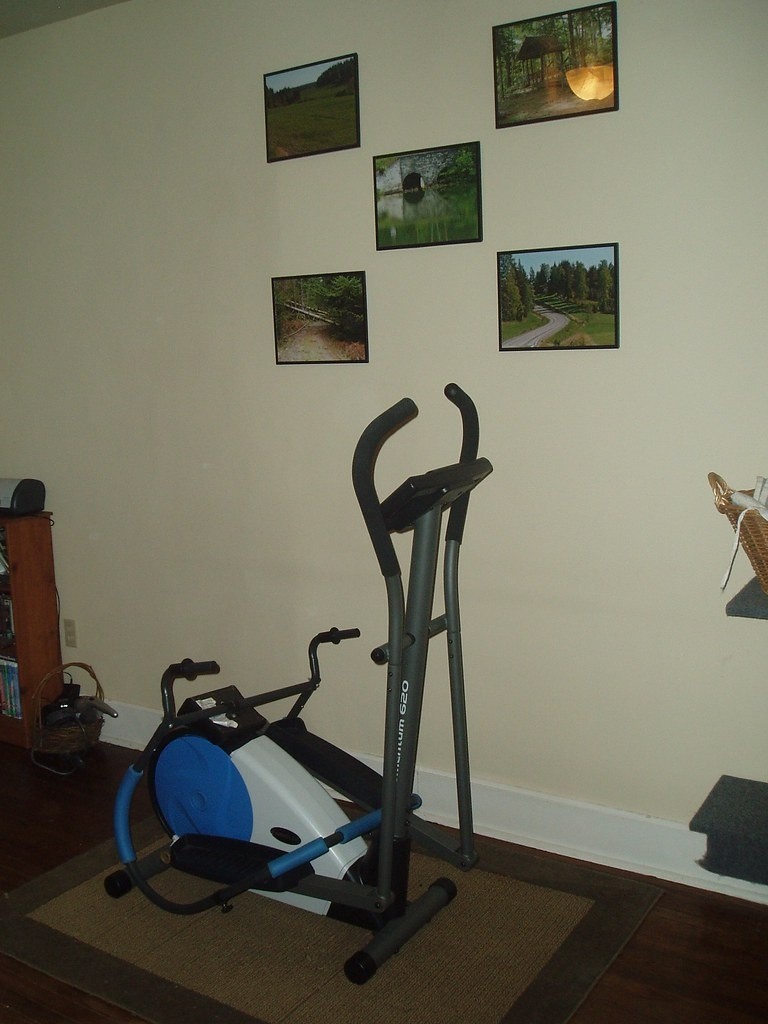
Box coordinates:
[0,805,665,1024]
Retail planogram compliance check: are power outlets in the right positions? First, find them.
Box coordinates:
[64,619,78,647]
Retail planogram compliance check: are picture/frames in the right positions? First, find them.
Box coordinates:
[496,241,620,351]
[372,141,483,251]
[490,2,620,129]
[271,270,369,365]
[264,52,360,164]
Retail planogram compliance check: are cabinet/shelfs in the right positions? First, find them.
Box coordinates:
[0,512,66,749]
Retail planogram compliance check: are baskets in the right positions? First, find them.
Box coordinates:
[29,662,105,754]
